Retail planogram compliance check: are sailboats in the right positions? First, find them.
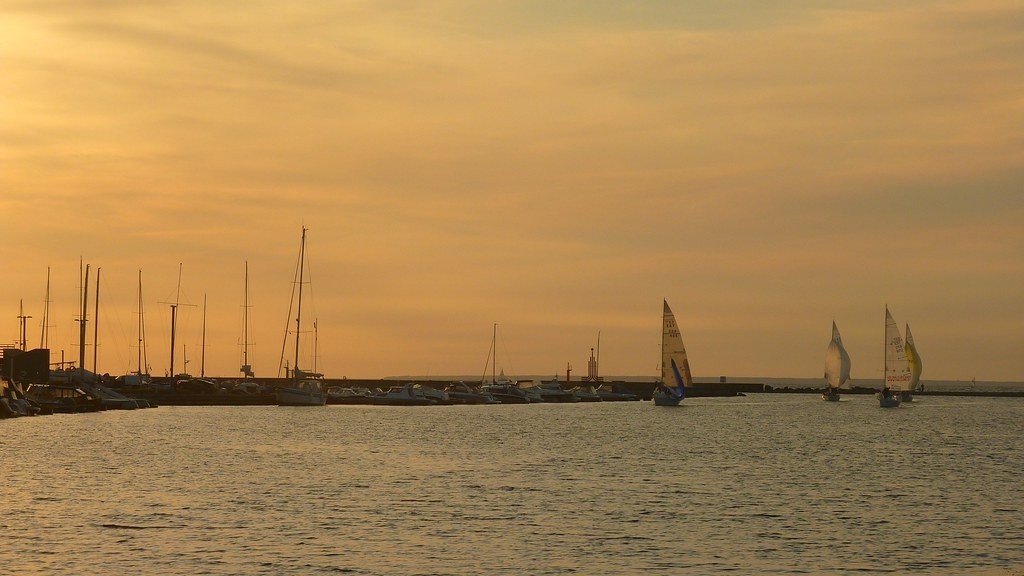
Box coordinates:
[821,319,852,401]
[878,304,913,407]
[904,323,923,402]
[653,298,693,406]
[1,224,643,421]
